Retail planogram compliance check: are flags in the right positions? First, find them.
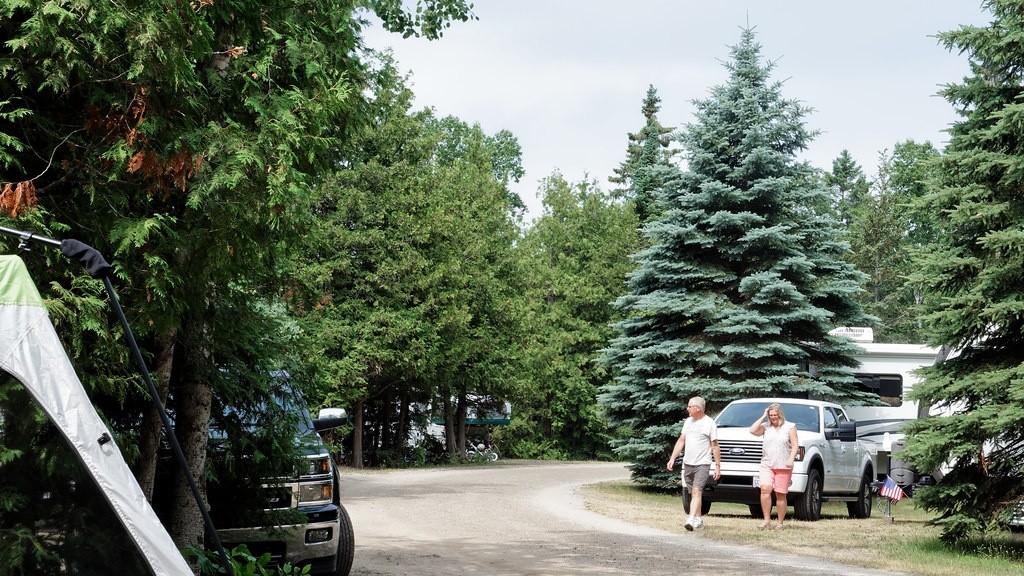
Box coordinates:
[880,475,903,502]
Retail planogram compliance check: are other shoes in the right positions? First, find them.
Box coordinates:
[757,523,770,530]
[775,524,784,531]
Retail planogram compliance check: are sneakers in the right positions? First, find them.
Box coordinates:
[693,520,705,531]
[685,519,693,531]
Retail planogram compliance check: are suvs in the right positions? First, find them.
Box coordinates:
[123,365,355,576]
[682,398,875,523]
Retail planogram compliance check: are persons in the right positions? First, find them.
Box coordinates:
[667,396,720,532]
[749,403,799,531]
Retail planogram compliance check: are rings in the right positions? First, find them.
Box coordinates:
[718,477,719,479]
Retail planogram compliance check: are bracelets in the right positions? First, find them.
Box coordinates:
[715,464,720,468]
